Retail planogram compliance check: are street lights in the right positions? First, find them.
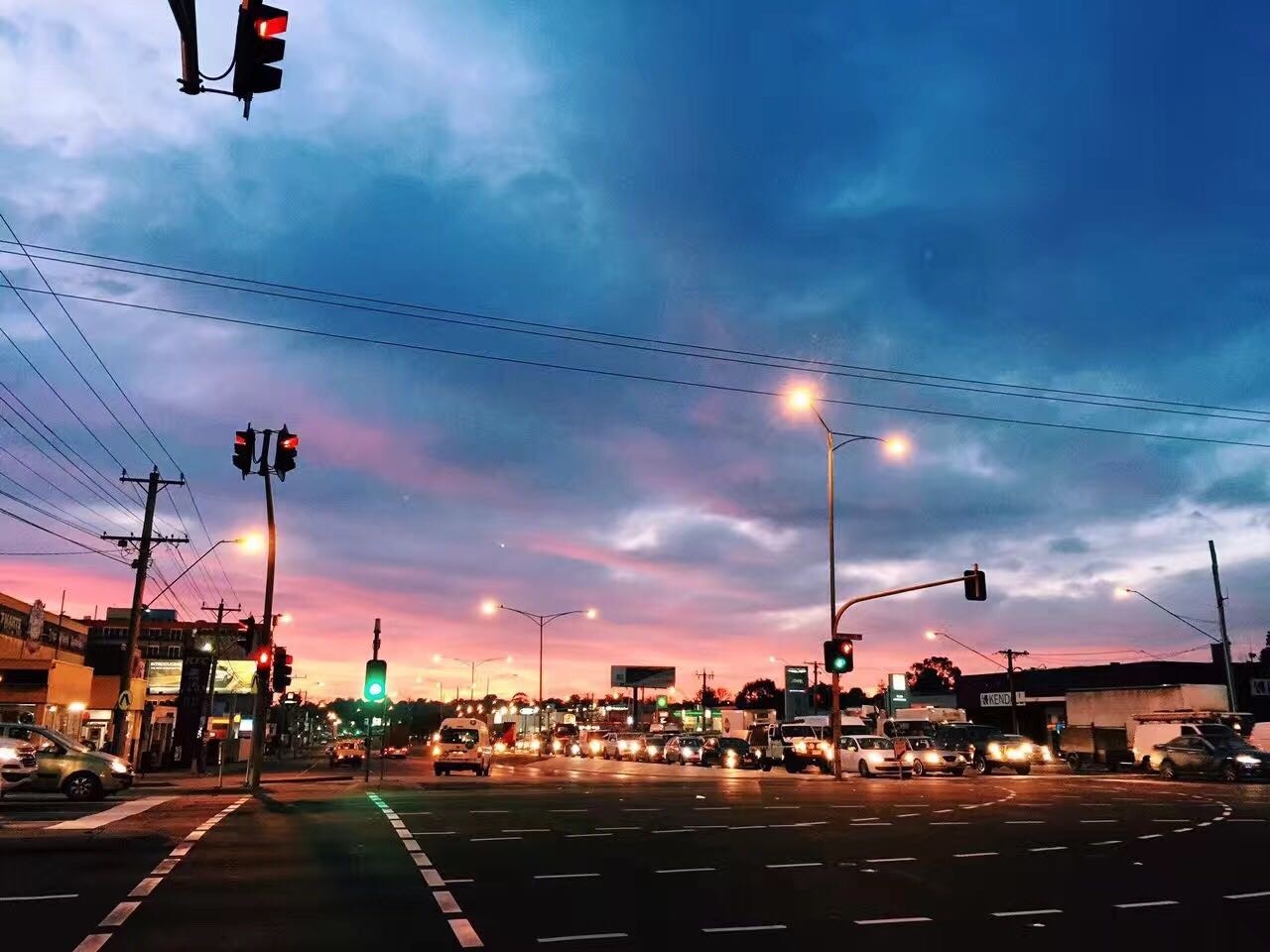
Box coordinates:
[109,533,264,764]
[1114,586,1237,713]
[788,386,908,781]
[433,654,512,702]
[482,598,598,761]
[926,632,1020,736]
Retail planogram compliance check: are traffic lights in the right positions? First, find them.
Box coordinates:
[823,640,854,673]
[364,657,387,700]
[237,615,254,654]
[272,647,293,693]
[231,428,256,477]
[257,647,271,667]
[232,2,287,101]
[273,421,299,482]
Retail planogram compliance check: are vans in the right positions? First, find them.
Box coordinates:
[431,718,490,777]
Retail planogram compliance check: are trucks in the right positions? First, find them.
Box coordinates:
[1054,722,1136,772]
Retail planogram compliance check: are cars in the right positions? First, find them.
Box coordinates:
[612,730,758,768]
[758,705,1050,779]
[488,726,611,759]
[369,743,411,759]
[314,731,332,745]
[1,723,136,804]
[333,734,363,766]
[1132,724,1270,784]
